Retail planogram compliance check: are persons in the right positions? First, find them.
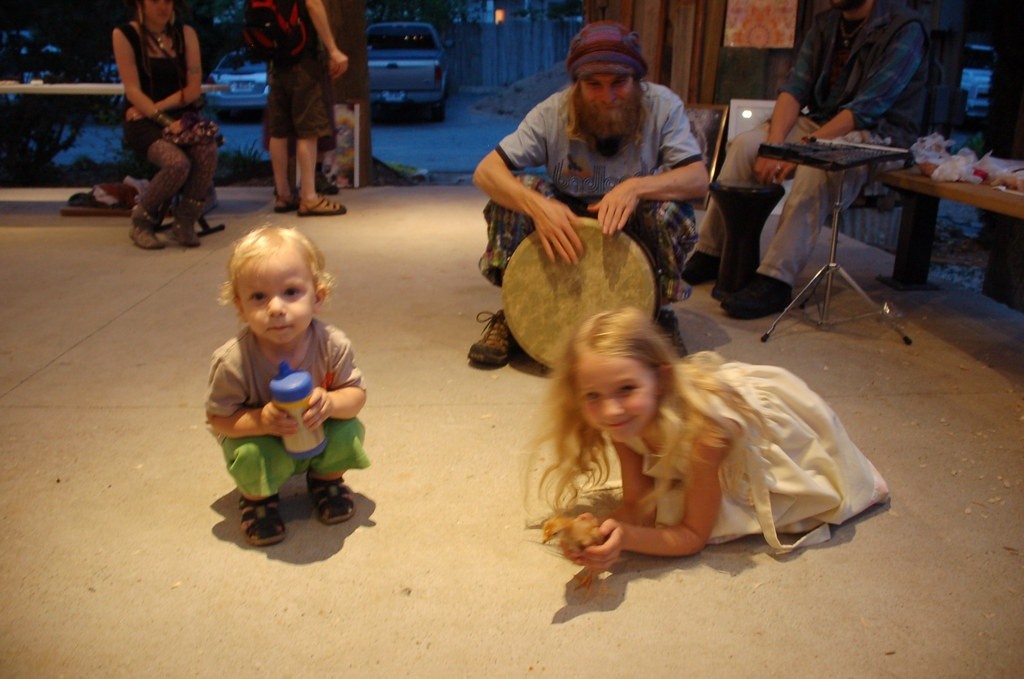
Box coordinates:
[266,0,349,216]
[113,0,218,250]
[468,22,711,366]
[524,305,889,571]
[679,0,934,318]
[205,225,370,547]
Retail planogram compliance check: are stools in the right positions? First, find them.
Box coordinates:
[125,175,226,238]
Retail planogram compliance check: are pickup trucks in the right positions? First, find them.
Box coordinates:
[364,22,461,124]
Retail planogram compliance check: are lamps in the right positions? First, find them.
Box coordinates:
[495,8,506,27]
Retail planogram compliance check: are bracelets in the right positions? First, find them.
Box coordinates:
[151,110,176,127]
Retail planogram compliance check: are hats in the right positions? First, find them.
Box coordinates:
[565,19,648,80]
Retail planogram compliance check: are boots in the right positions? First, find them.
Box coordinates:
[129,206,164,249]
[171,196,207,246]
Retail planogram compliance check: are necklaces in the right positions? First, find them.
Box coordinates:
[146,29,167,50]
[841,11,870,46]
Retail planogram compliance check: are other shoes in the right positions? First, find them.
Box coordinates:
[720,273,793,321]
[680,250,721,285]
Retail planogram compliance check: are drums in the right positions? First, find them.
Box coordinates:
[708,182,785,302]
[503,211,662,369]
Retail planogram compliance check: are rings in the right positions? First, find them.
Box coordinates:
[777,166,783,173]
[133,113,137,116]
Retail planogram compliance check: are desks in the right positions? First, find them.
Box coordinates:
[0,83,230,94]
[876,167,1024,293]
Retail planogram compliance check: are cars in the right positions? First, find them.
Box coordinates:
[207,50,269,113]
[959,45,994,119]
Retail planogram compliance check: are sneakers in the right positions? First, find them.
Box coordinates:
[468,309,510,367]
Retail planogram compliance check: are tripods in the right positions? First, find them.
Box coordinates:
[757,139,913,346]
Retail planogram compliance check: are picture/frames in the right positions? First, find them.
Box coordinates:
[720,0,804,57]
[685,104,729,211]
[729,99,809,215]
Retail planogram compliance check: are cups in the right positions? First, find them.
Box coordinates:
[268,364,329,460]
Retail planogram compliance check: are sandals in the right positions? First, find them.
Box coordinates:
[239,494,287,546]
[306,472,354,525]
[297,194,346,215]
[275,192,301,213]
